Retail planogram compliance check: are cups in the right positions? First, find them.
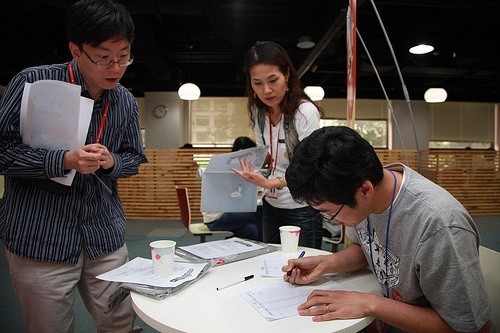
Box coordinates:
[150,239,176,276]
[278,226,300,252]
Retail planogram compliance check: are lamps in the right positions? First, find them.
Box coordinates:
[424,88,447,103]
[297,34,315,48]
[178,82,200,101]
[304,86,324,101]
[409,36,436,56]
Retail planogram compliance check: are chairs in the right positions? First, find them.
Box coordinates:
[477,246,500,333]
[321,224,347,251]
[175,187,233,243]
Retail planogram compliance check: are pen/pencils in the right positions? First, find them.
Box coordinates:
[216,275,255,291]
[287,250,306,276]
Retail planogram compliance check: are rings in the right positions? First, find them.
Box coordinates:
[326,305,329,312]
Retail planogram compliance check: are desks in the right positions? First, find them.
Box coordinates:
[129,239,390,333]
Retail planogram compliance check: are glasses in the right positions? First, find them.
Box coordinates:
[78,46,135,68]
[319,204,344,221]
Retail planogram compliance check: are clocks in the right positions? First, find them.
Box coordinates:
[152,104,169,119]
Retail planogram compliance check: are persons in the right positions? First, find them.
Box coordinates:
[178,137,264,243]
[0,0,149,333]
[282,126,492,333]
[229,41,324,250]
